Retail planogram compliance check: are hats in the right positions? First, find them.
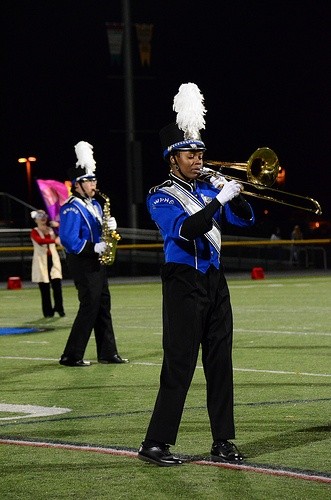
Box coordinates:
[71,141,97,182]
[163,82,207,156]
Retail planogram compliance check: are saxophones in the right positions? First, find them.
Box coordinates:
[92,188,122,266]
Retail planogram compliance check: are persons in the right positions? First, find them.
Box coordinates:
[137,120,256,465]
[57,165,129,366]
[269,226,308,260]
[30,210,65,317]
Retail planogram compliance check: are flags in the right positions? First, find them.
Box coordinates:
[37,179,73,233]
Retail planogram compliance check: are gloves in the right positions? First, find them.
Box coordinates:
[210,173,229,190]
[55,237,61,246]
[50,221,59,227]
[216,180,244,206]
[107,217,117,231]
[94,242,107,256]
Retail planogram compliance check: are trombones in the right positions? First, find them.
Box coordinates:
[197,147,322,215]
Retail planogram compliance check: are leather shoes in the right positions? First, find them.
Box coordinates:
[101,354,130,364]
[60,359,91,366]
[138,442,183,466]
[211,440,244,463]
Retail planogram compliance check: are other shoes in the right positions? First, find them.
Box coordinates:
[49,309,54,317]
[54,306,65,317]
